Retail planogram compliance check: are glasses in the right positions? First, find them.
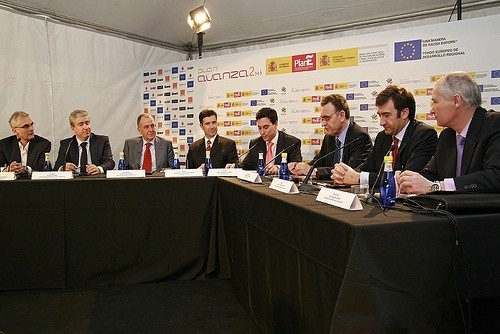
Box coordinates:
[319,110,339,122]
[14,122,34,130]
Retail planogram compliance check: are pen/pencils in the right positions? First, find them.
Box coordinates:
[75,167,87,169]
[398,166,403,196]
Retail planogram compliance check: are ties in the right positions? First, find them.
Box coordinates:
[266,142,274,172]
[392,137,399,170]
[142,142,152,173]
[80,142,88,174]
[206,140,211,150]
[333,140,341,167]
[456,135,465,177]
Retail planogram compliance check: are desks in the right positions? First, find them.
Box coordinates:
[0,166,500,334]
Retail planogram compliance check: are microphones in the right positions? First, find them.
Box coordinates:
[258,140,300,181]
[298,136,364,190]
[63,138,74,170]
[7,137,21,172]
[363,145,395,203]
[235,140,259,168]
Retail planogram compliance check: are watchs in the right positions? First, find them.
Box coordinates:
[429,180,441,191]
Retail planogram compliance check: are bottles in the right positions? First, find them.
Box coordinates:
[172,150,180,169]
[257,152,266,177]
[379,155,396,207]
[279,153,289,181]
[205,150,212,177]
[43,153,52,171]
[118,151,126,170]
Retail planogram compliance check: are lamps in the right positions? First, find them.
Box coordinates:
[186,5,212,58]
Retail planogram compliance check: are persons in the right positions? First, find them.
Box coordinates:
[225,107,302,174]
[393,71,500,193]
[0,110,52,174]
[53,109,115,175]
[331,85,437,187]
[287,94,373,178]
[186,110,239,169]
[123,113,174,173]
